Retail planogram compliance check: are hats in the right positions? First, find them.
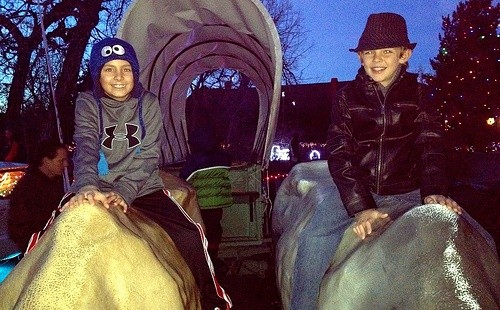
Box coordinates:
[350,12,418,57]
[87,38,147,144]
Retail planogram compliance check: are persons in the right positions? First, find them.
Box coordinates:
[288,10,500,310]
[8,138,70,257]
[180,129,232,259]
[25,37,232,310]
[289,131,301,164]
[1,127,29,163]
